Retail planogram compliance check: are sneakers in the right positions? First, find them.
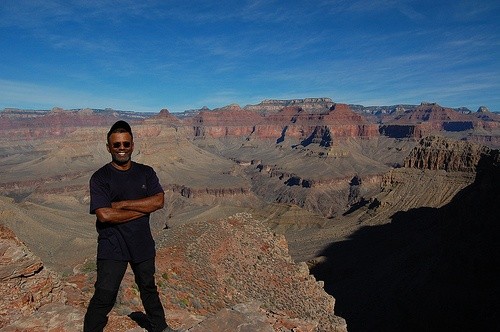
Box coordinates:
[160,323,187,332]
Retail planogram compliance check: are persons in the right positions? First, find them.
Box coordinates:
[84,119,179,332]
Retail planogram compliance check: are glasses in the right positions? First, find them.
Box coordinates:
[109,141,133,148]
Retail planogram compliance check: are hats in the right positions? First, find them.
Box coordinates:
[107,120,132,134]
